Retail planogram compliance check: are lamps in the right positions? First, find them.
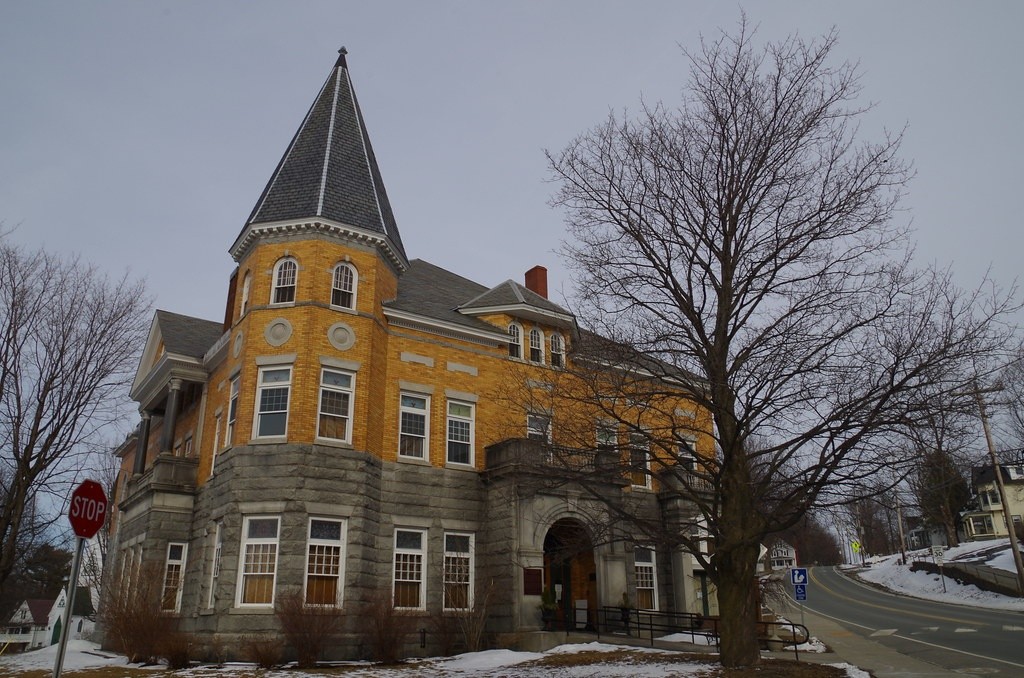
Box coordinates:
[285,249,289,257]
[344,254,350,262]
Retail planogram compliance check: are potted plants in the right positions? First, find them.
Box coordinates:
[535,591,572,631]
[620,592,634,624]
